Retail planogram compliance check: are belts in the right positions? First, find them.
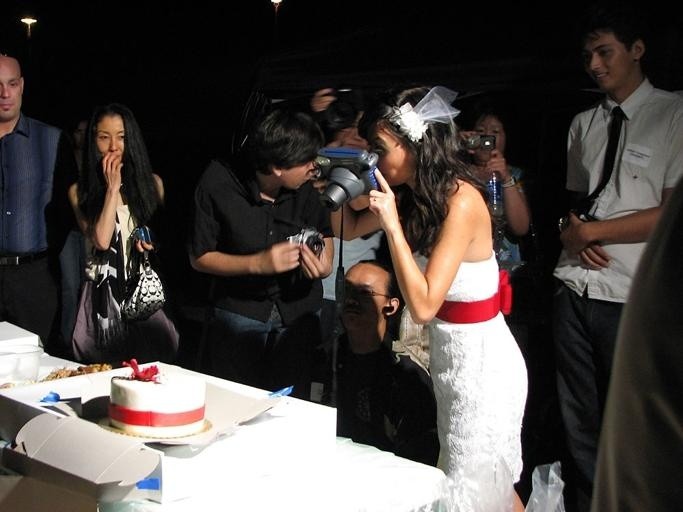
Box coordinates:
[1,250,50,265]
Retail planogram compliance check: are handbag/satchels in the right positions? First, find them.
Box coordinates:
[121,266,165,321]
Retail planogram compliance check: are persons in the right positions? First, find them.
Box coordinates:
[68,105,187,365]
[306,78,365,149]
[540,21,683,512]
[462,108,535,309]
[361,85,536,511]
[187,111,338,402]
[334,259,436,466]
[591,168,683,512]
[0,55,78,335]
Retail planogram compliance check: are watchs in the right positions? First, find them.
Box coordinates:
[499,173,518,192]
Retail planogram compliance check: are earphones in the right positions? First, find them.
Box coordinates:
[382,306,394,313]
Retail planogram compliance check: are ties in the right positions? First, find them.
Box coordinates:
[572,106,626,210]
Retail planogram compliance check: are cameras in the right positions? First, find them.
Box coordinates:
[466,134,496,151]
[324,79,368,129]
[130,225,151,247]
[284,226,325,258]
[313,146,385,212]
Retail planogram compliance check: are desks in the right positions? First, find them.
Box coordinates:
[0,320,456,512]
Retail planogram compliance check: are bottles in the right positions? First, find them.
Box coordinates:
[483,163,503,216]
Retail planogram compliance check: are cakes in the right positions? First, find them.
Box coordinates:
[107,359,207,439]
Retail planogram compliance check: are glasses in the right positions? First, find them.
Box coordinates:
[344,287,391,298]
